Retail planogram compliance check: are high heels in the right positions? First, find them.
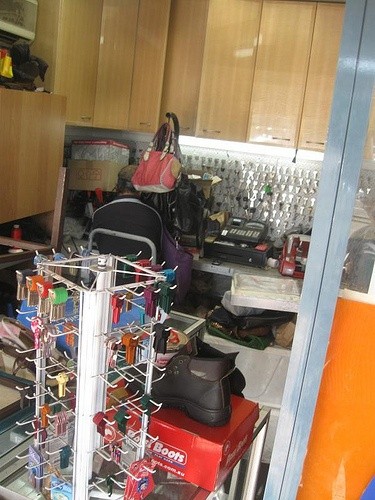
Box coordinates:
[0,314,68,376]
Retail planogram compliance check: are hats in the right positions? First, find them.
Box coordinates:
[118,164,140,181]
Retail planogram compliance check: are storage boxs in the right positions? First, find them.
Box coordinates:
[71,381,260,493]
[134,328,191,365]
[67,141,131,196]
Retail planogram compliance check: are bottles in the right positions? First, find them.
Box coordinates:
[11,225,21,249]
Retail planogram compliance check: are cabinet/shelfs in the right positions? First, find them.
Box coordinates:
[1,1,374,499]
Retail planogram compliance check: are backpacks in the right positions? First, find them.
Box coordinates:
[172,173,207,249]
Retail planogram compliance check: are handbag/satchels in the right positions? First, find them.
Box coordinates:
[280,234,312,278]
[206,305,295,349]
[138,123,173,231]
[131,122,181,192]
[161,223,193,305]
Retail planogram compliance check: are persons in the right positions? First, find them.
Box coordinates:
[90,164,166,262]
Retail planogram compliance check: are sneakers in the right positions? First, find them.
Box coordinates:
[126,336,240,425]
